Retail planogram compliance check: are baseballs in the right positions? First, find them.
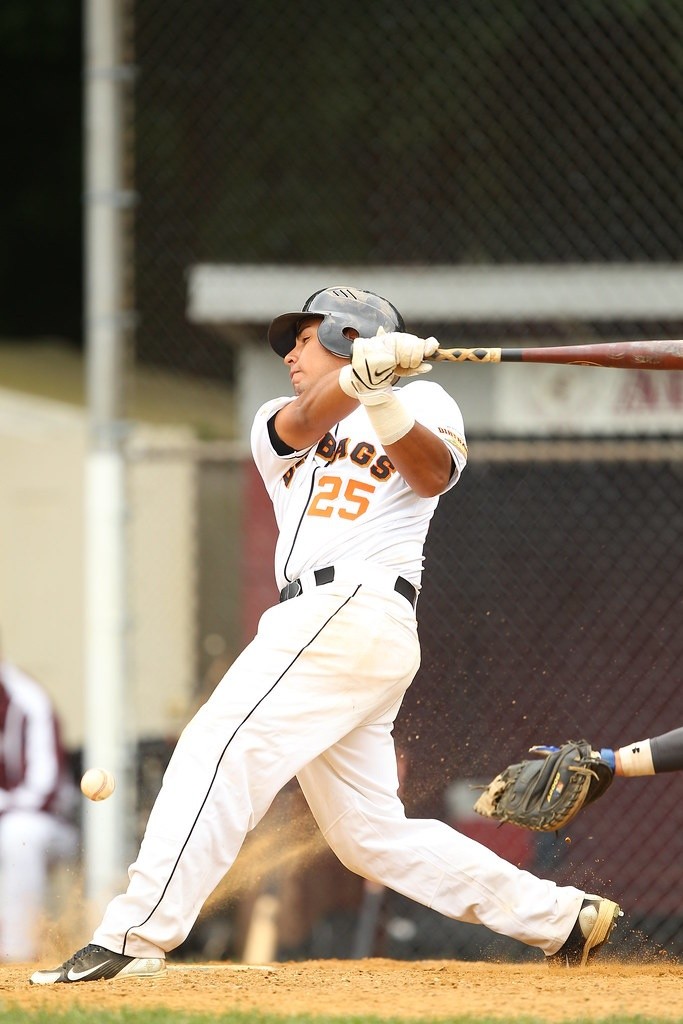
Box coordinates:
[78,766,119,804]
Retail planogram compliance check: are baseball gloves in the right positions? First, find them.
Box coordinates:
[470,735,620,838]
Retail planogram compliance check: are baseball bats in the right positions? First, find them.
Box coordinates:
[344,335,683,377]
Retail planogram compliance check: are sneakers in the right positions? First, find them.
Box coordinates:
[546,893,621,968]
[29,944,170,986]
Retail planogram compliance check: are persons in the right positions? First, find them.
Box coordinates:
[474,727,683,831]
[0,666,81,966]
[27,287,624,984]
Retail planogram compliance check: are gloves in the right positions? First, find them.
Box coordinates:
[338,325,440,407]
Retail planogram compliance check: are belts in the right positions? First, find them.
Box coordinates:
[279,564,417,611]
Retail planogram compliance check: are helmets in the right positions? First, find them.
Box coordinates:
[267,285,409,360]
